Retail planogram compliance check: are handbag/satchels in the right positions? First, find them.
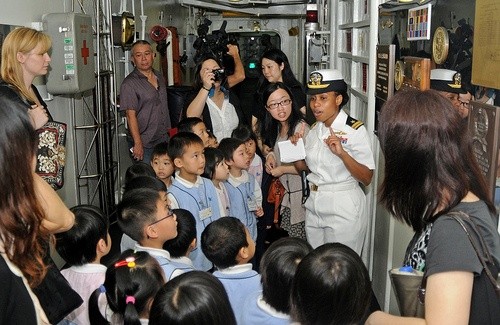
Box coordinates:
[267,176,285,223]
[0,83,67,191]
[389,211,500,325]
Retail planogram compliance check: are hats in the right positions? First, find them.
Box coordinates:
[305,69,347,94]
[430,69,467,94]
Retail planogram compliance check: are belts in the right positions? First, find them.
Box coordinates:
[308,180,359,192]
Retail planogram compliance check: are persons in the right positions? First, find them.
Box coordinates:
[2,27,66,186]
[120,42,173,164]
[261,82,311,244]
[288,69,375,258]
[365,89,500,324]
[183,43,246,144]
[252,51,307,152]
[0,116,374,324]
[428,69,497,124]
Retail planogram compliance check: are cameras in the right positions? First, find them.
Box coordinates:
[210,69,225,81]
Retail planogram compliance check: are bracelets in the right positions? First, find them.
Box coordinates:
[202,87,210,92]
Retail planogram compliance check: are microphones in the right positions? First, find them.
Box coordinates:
[216,20,227,44]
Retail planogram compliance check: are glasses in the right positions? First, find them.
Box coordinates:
[458,100,469,109]
[266,99,291,110]
[149,209,173,227]
